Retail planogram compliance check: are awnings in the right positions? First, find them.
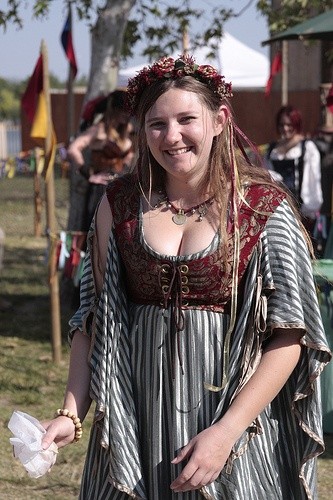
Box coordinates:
[261,9,333,46]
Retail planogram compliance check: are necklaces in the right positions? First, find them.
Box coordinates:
[154,177,226,225]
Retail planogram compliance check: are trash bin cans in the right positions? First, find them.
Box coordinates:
[310,259,333,433]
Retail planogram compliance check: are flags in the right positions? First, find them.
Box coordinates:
[60,11,78,81]
[20,54,50,151]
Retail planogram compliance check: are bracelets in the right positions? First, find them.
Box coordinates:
[53,408,82,443]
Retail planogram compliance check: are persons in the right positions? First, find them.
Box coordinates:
[64,89,137,234]
[263,103,324,240]
[42,52,333,500]
